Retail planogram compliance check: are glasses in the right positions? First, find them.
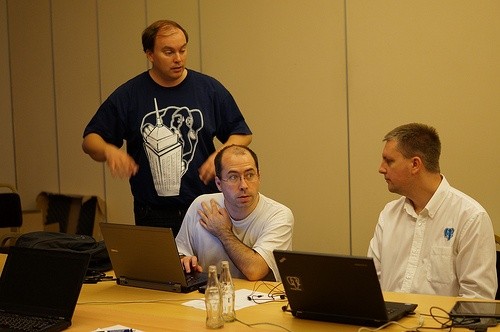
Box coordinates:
[218,171,259,183]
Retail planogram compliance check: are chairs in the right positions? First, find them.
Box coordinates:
[0,192,22,243]
[36,193,107,240]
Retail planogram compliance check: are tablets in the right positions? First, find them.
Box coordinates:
[449,300,500,318]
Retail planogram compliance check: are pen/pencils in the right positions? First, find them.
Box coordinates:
[247,294,286,299]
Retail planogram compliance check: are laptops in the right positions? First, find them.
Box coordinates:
[99,222,221,294]
[0,246,93,332]
[272,250,418,328]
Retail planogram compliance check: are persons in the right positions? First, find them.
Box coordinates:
[367,123,497,299]
[82,19,253,239]
[175,144,294,282]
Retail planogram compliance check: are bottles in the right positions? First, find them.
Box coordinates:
[219,261,236,322]
[205,264,224,329]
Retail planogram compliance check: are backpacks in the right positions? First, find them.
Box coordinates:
[11,231,113,273]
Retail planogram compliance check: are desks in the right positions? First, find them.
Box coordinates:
[0,253,500,332]
